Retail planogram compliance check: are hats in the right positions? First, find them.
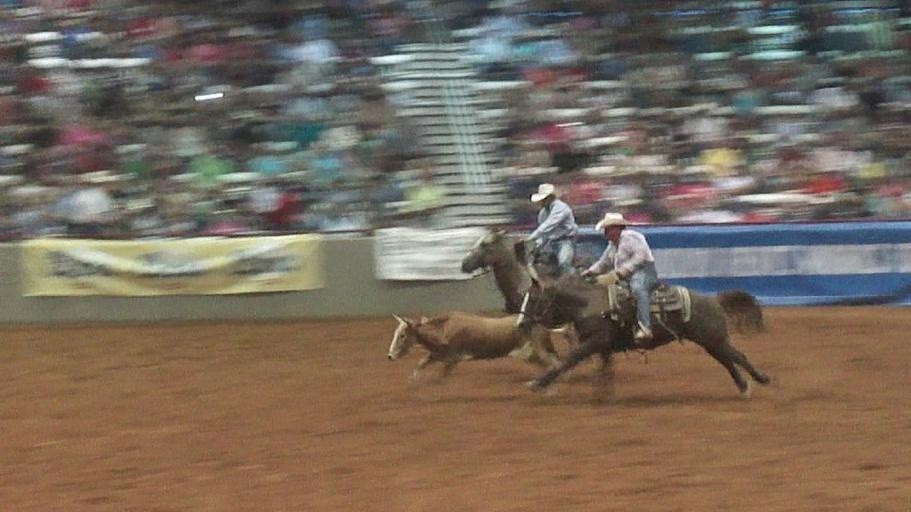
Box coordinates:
[594,212,633,232]
[531,183,562,203]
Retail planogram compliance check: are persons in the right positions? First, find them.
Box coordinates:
[524,182,580,277]
[2,0,911,233]
[583,213,658,340]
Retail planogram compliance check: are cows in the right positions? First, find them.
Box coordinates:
[388,312,563,384]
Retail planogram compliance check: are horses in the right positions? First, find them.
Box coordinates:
[461,226,619,371]
[516,267,771,400]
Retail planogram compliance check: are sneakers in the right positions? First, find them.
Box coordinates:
[634,327,654,340]
